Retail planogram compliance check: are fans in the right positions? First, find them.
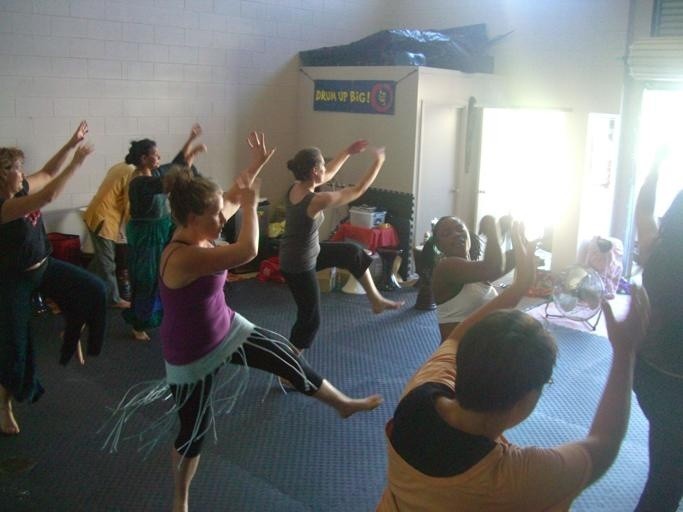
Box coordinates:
[543,264,607,331]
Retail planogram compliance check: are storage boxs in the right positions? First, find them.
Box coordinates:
[348,209,384,230]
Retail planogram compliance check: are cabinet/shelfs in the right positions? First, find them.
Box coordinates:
[223,198,273,274]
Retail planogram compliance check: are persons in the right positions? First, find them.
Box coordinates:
[0,121,108,437]
[434,214,513,343]
[372,221,649,511]
[124,126,209,341]
[157,131,384,512]
[631,161,683,511]
[280,138,407,392]
[84,164,137,307]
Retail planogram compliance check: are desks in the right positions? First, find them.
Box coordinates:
[337,222,397,295]
